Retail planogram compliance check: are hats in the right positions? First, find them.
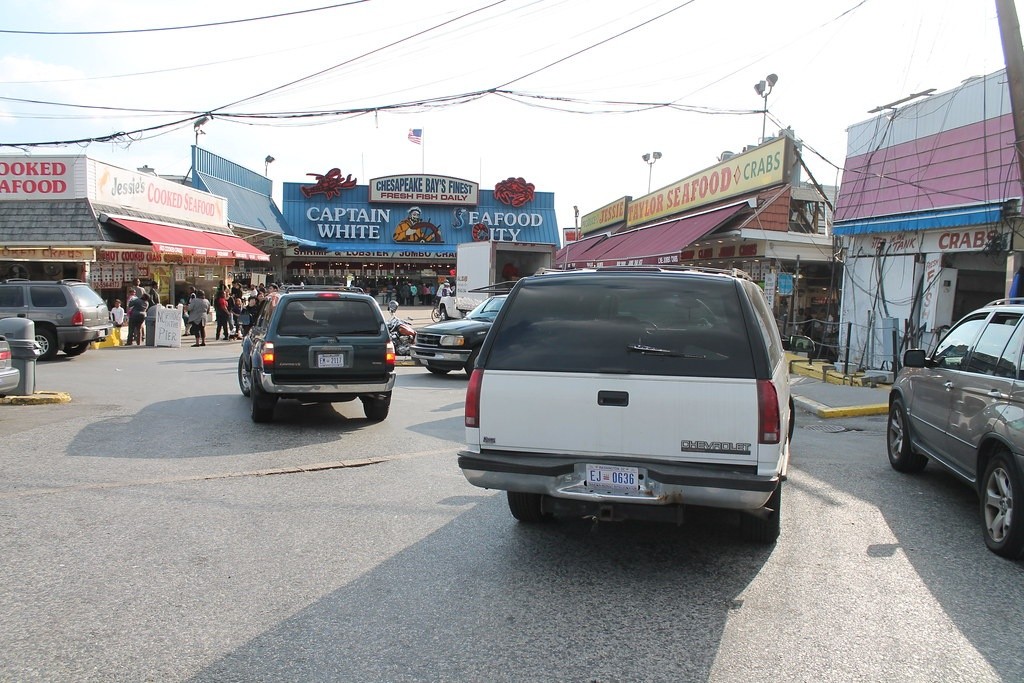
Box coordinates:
[444,283,450,287]
[445,280,449,282]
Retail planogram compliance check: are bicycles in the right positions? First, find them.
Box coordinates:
[430,296,441,323]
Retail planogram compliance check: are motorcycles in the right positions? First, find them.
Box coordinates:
[386,301,417,356]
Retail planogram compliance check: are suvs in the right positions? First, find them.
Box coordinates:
[887,298,1024,558]
[457,265,816,543]
[409,281,520,377]
[0,279,113,360]
[238,286,397,423]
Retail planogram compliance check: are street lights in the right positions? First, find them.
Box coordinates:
[753,74,778,144]
[642,152,662,194]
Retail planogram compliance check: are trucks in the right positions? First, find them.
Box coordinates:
[439,240,557,321]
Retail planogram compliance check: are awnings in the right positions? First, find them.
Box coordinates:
[110,218,271,262]
[554,203,747,269]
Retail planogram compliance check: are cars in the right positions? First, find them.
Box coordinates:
[0,335,20,398]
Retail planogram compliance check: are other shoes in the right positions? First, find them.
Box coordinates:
[223,337,228,340]
[215,337,220,340]
[191,344,199,347]
[183,333,189,336]
[125,343,132,345]
[199,344,206,346]
[136,340,140,345]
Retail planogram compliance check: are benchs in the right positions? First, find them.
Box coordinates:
[522,323,748,360]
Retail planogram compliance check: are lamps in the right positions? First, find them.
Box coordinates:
[265,155,275,176]
[194,116,208,145]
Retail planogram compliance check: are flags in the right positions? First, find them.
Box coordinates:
[408,129,422,144]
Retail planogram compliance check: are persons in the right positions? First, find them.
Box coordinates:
[385,280,456,308]
[110,299,124,327]
[124,278,160,346]
[214,280,279,340]
[182,286,210,346]
